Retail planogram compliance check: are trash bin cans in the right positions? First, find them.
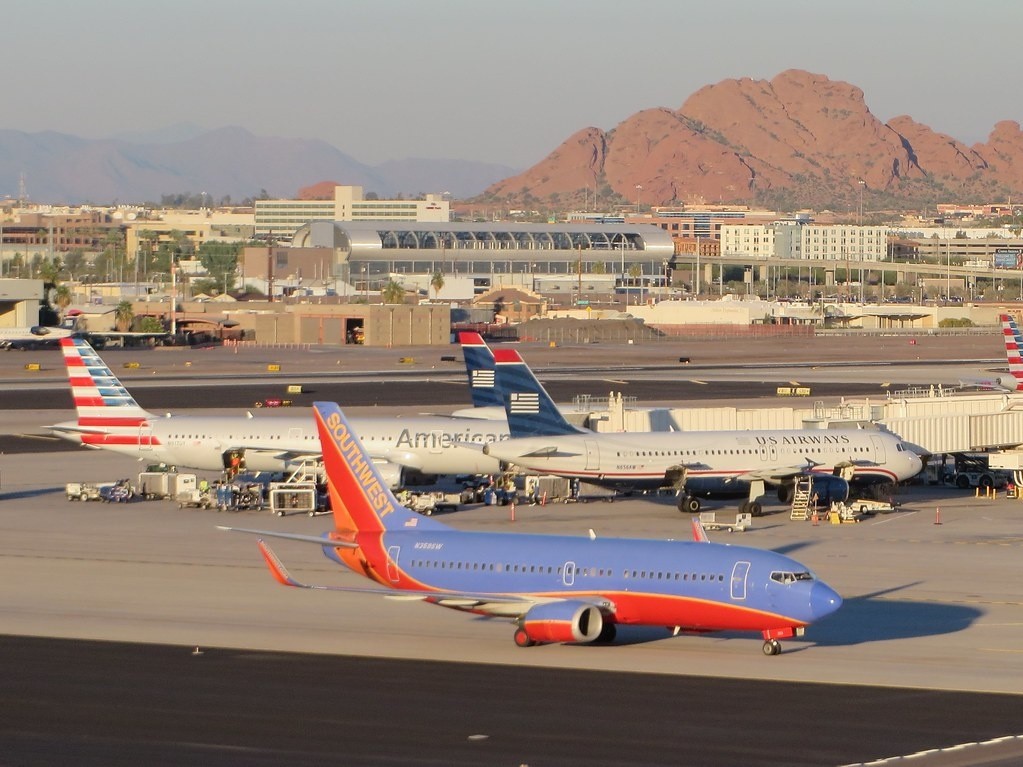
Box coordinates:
[95,297,102,305]
[1007,484,1015,498]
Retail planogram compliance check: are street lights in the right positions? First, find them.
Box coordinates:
[859,181,866,227]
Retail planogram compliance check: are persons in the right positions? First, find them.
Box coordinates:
[231,452,240,473]
[528,481,540,506]
[401,489,408,502]
[199,478,207,492]
[122,478,130,503]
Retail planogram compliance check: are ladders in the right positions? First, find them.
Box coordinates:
[790,475,814,521]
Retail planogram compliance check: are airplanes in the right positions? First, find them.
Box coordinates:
[38,333,924,517]
[214,401,847,649]
